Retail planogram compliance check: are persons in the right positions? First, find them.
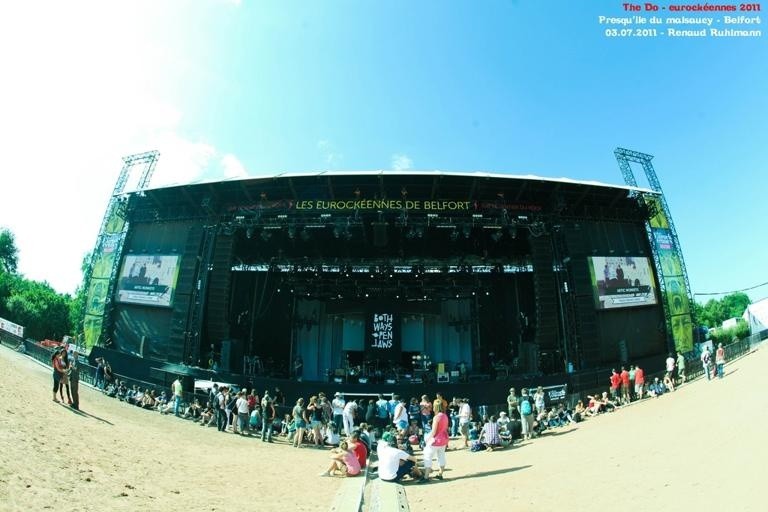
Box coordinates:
[139,263,147,277]
[50,342,729,484]
[615,264,624,280]
[603,264,610,283]
[129,263,135,277]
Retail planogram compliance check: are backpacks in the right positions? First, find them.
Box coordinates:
[519,397,533,416]
[212,392,222,410]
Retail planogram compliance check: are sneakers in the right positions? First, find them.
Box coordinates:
[416,472,430,484]
[318,471,332,477]
[52,399,80,410]
[433,474,443,481]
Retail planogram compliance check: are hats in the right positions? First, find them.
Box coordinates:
[499,411,508,416]
